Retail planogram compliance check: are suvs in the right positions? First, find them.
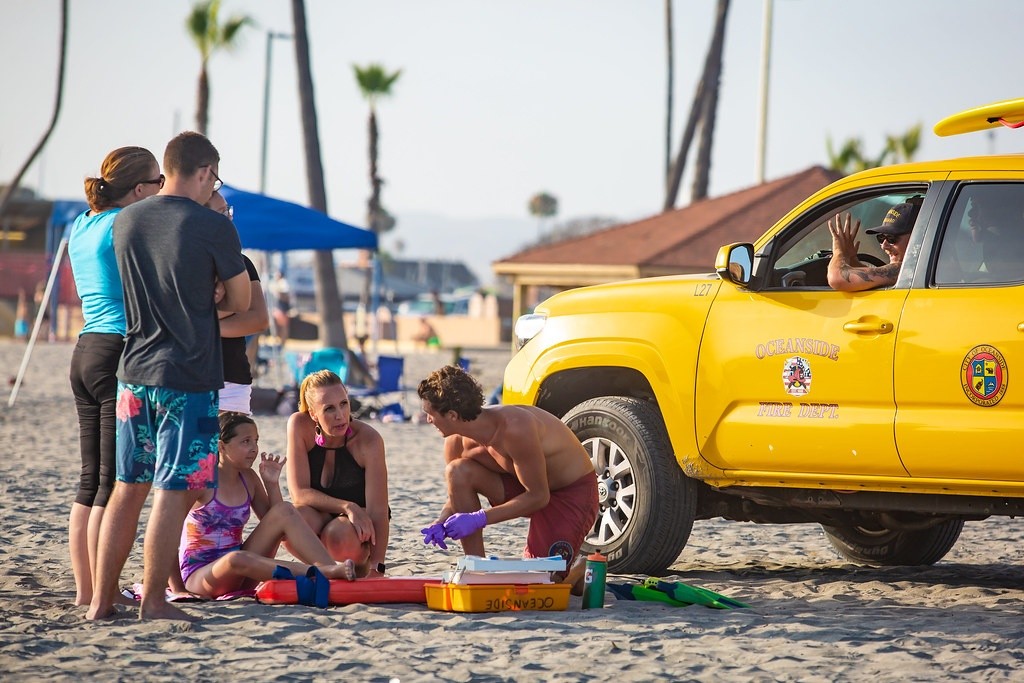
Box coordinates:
[501,93,1024,578]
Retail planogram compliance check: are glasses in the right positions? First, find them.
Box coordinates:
[197,165,223,192]
[129,174,165,190]
[219,205,234,218]
[876,232,908,244]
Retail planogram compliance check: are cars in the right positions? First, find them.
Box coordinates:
[283,254,496,317]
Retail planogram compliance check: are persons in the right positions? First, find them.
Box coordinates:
[418,364,600,596]
[84,131,252,620]
[274,271,291,348]
[411,318,440,353]
[14,280,49,340]
[826,203,921,291]
[203,191,268,417]
[68,147,165,606]
[167,412,356,598]
[430,291,444,316]
[967,188,1024,282]
[282,369,391,578]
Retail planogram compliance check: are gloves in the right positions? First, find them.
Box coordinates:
[420,523,448,550]
[443,509,486,540]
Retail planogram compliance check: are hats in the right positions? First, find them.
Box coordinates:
[864,203,918,235]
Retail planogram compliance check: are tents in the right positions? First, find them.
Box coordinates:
[8,185,383,411]
